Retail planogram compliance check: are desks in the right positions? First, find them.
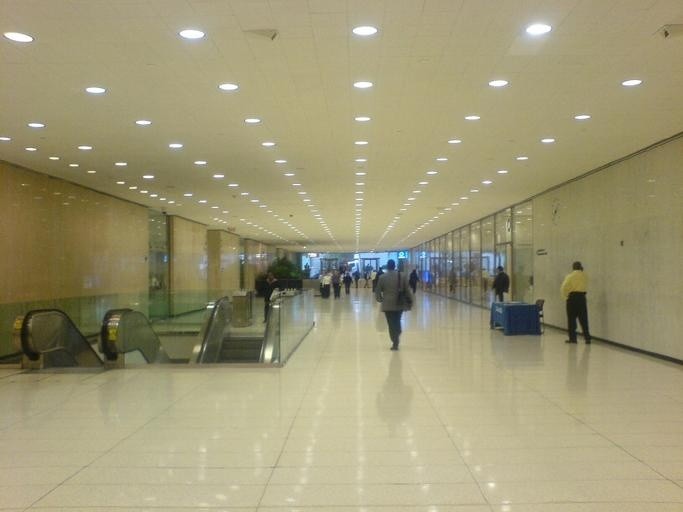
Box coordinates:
[492,301,540,336]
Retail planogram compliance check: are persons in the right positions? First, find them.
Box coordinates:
[260,270,286,324]
[558,260,593,345]
[490,265,511,303]
[408,268,420,294]
[446,266,457,296]
[481,267,488,294]
[373,258,413,351]
[317,261,384,301]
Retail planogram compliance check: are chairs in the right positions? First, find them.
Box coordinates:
[534,299,545,333]
